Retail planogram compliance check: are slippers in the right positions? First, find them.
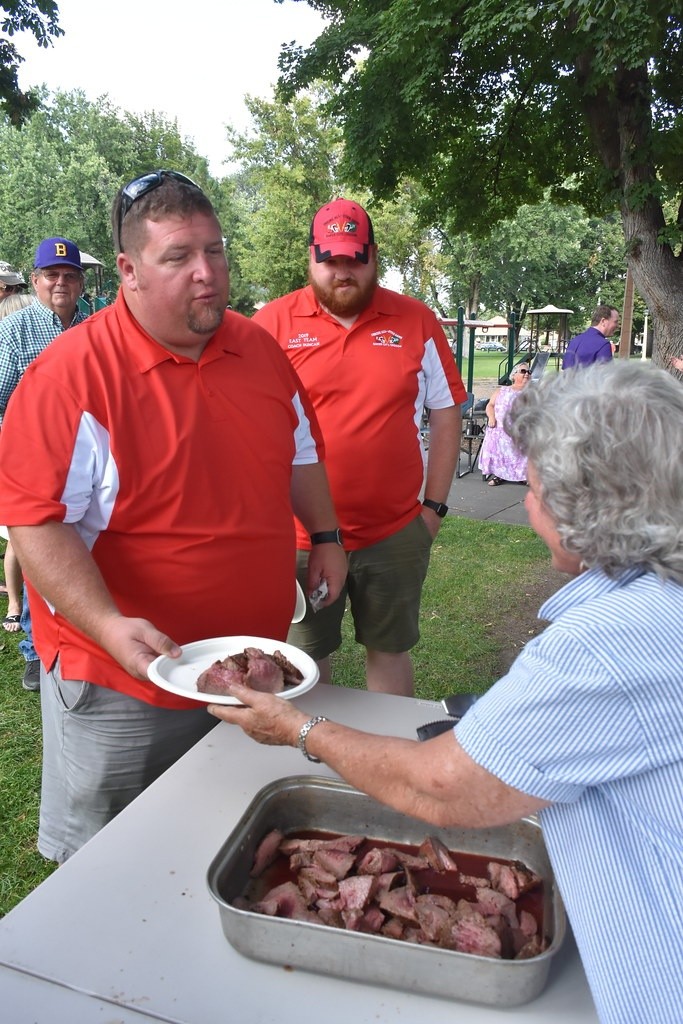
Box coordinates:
[0,580,24,597]
[2,611,22,632]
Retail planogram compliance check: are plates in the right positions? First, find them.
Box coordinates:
[147,635,320,706]
[292,578,307,623]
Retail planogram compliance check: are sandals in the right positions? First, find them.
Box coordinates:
[517,481,529,487]
[489,476,504,486]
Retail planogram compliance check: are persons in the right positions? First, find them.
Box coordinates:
[250,198,469,697]
[478,362,532,486]
[207,359,681,1024]
[562,305,620,371]
[0,169,348,863]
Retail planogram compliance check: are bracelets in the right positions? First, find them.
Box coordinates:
[297,716,328,764]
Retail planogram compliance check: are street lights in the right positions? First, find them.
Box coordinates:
[642,309,650,360]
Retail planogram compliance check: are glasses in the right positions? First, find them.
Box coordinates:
[117,170,203,253]
[38,271,81,283]
[0,287,20,294]
[516,369,532,374]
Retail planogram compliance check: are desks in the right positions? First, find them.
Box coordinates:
[1,681,602,1024]
[456,409,488,479]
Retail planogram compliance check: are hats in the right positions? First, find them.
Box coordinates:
[309,198,374,264]
[0,261,28,289]
[34,238,85,272]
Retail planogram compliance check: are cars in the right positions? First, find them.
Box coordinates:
[518,342,535,351]
[481,342,507,352]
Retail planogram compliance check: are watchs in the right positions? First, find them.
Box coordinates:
[421,499,448,518]
[309,528,343,546]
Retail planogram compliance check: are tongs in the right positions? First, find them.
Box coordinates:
[416,695,484,741]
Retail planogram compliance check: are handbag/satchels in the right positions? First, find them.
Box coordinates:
[463,424,485,436]
